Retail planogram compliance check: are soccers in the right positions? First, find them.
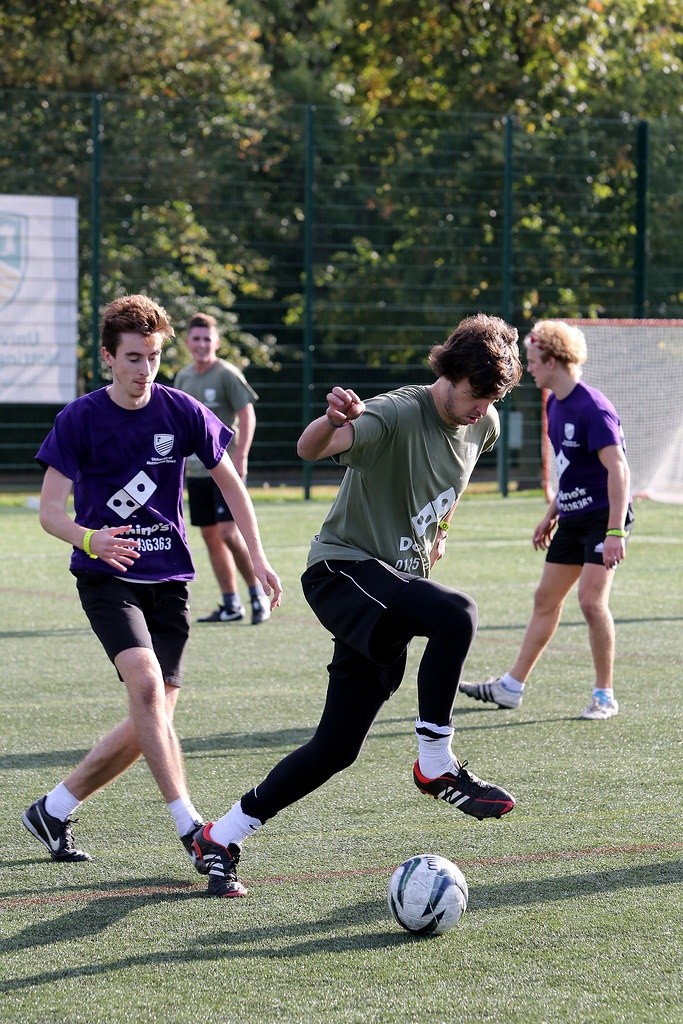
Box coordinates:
[386,853,471,938]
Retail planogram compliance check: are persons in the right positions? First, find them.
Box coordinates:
[21,294,283,875]
[458,318,635,720]
[191,312,523,898]
[173,312,272,624]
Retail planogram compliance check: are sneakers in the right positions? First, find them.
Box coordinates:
[180,818,209,874]
[192,822,247,897]
[250,595,271,624]
[197,604,245,623]
[459,677,523,710]
[579,690,620,720]
[22,795,92,862]
[413,758,516,820]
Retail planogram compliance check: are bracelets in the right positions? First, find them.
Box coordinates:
[439,520,453,531]
[83,530,98,560]
[606,529,629,537]
